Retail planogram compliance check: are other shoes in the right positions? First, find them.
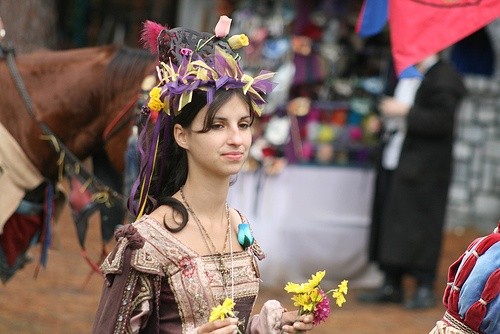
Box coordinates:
[359,289,402,302]
[407,294,435,310]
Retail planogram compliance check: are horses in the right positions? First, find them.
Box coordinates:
[0,37,161,290]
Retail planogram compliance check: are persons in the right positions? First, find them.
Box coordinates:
[359,44,458,311]
[212,1,394,170]
[427,218,500,334]
[101,72,314,334]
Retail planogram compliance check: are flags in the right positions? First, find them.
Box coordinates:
[354,0,499,81]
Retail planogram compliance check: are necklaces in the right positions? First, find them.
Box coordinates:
[180,183,243,334]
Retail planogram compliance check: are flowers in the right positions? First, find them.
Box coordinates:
[197,15,249,52]
[148,86,166,111]
[208,297,243,334]
[284,269,348,327]
[237,224,254,251]
